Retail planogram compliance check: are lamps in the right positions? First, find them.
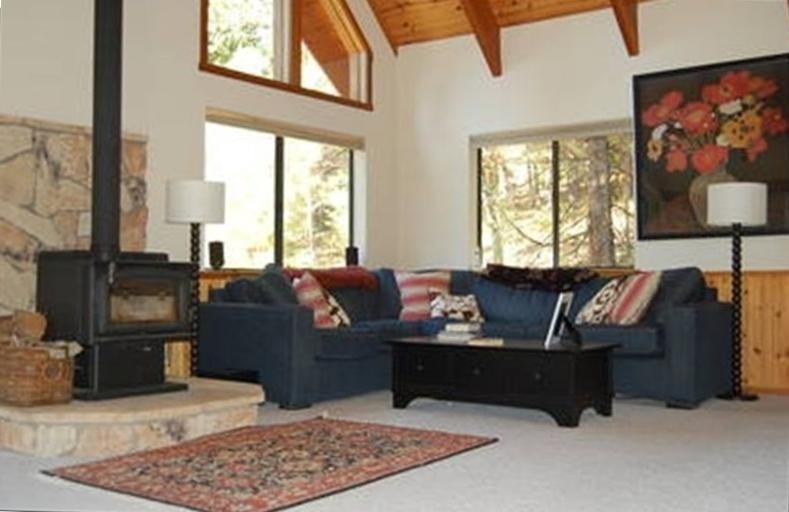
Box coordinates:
[165,179,225,380]
[707,181,769,402]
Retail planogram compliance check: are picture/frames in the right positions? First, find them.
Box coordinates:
[545,292,582,347]
[631,52,789,239]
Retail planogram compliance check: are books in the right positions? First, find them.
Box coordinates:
[434,319,505,348]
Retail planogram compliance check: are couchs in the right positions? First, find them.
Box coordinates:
[197,267,735,409]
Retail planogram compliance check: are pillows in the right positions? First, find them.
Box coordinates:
[428,288,486,324]
[605,269,663,327]
[292,272,334,330]
[575,274,627,326]
[260,263,295,305]
[394,269,451,320]
[227,277,259,303]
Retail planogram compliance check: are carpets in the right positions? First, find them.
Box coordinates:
[39,417,498,512]
